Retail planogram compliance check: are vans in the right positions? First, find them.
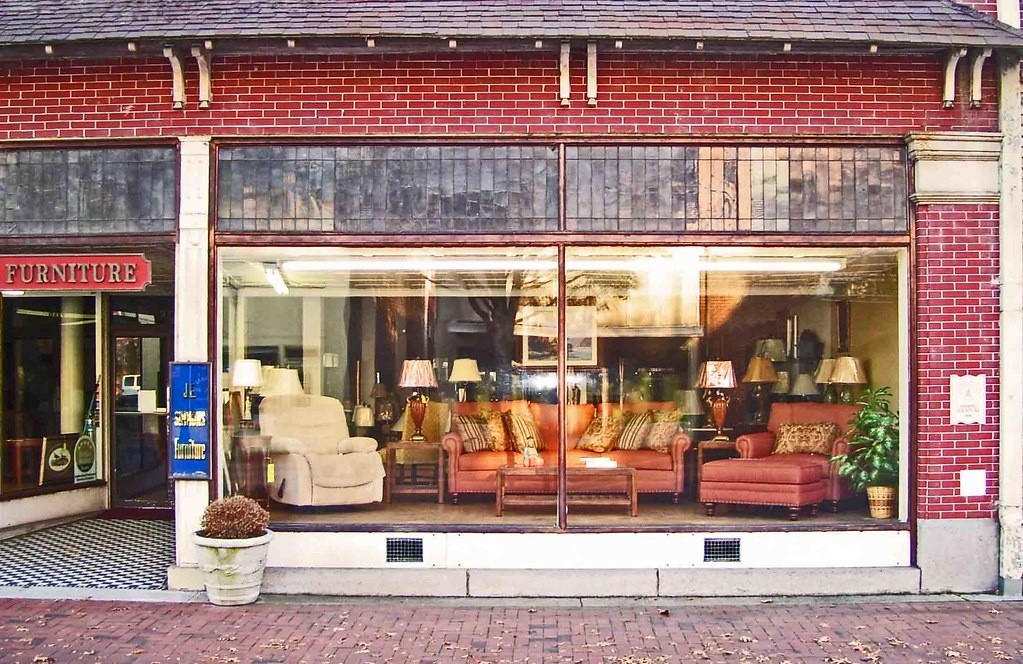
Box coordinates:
[119,374,141,407]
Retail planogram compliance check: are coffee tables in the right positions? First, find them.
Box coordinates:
[491,464,637,518]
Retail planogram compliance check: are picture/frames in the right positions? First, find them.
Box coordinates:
[38,434,83,487]
[522,306,598,368]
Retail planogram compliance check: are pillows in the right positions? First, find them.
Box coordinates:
[615,412,652,451]
[503,409,549,454]
[455,413,491,452]
[641,409,682,454]
[574,415,626,453]
[479,408,515,454]
[775,421,837,456]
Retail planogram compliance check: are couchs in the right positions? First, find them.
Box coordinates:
[700,403,867,523]
[442,400,692,504]
[258,393,386,515]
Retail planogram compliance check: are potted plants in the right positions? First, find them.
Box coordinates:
[828,385,898,517]
[193,494,274,607]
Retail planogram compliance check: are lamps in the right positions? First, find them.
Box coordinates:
[672,339,791,442]
[233,358,305,431]
[831,357,868,402]
[264,263,288,294]
[398,358,439,441]
[788,373,821,403]
[815,358,837,402]
[350,383,398,445]
[448,358,481,403]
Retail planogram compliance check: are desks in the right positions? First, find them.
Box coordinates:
[696,441,737,506]
[4,437,42,486]
[231,434,272,513]
[385,441,447,503]
